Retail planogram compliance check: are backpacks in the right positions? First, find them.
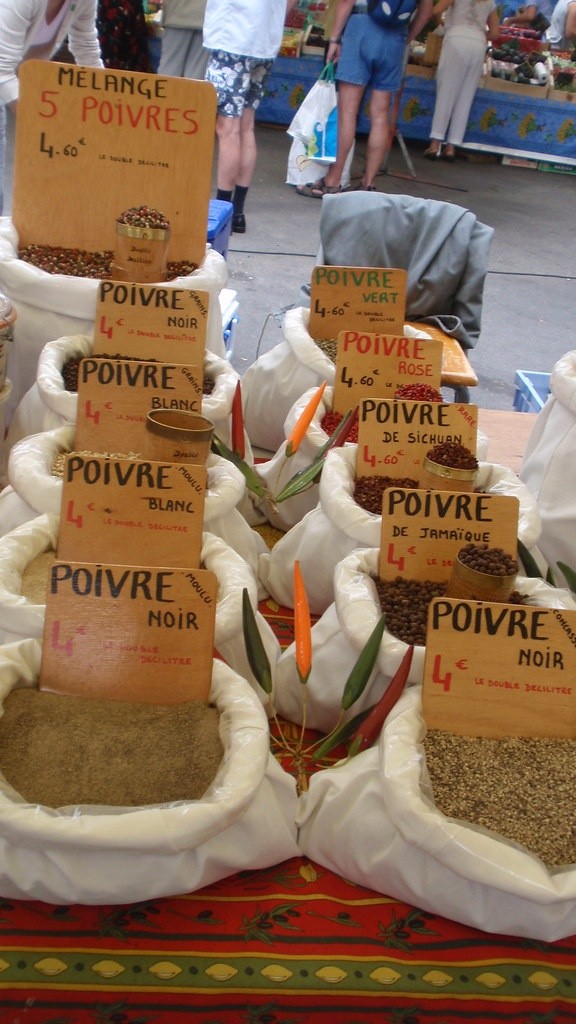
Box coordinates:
[367,0,418,28]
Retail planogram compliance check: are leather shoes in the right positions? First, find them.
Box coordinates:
[232,214,245,233]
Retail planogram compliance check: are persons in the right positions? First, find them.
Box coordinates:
[296,0,433,199]
[0,0,297,235]
[422,0,500,163]
[501,0,576,51]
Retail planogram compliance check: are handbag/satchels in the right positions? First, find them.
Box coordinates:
[285,138,356,190]
[424,31,443,65]
[286,61,338,166]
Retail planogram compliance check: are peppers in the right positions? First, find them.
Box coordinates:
[285,381,327,456]
[210,379,244,460]
[320,405,360,456]
[242,558,414,759]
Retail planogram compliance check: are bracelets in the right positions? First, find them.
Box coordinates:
[328,39,339,43]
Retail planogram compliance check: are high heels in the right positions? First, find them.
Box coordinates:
[423,148,438,160]
[441,150,454,164]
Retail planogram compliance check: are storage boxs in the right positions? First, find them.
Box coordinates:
[283,0,576,104]
[212,287,240,362]
[203,197,237,263]
[512,370,551,412]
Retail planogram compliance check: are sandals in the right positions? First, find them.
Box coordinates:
[297,177,341,199]
[343,186,375,194]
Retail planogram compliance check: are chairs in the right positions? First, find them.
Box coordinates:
[303,187,497,406]
